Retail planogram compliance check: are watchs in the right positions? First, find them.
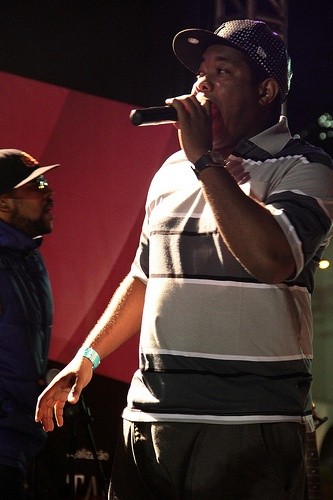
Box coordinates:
[190,148,227,181]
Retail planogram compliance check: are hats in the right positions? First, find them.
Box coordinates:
[0,148,61,195]
[172,19,294,104]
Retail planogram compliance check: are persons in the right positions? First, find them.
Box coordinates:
[34,18,333,500]
[0,148,55,500]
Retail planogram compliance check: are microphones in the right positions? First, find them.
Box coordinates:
[130,105,178,127]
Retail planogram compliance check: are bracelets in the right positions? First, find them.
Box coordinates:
[76,345,103,370]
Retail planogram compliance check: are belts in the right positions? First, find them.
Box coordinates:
[130,422,164,500]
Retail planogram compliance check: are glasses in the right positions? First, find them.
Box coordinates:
[8,175,49,195]
